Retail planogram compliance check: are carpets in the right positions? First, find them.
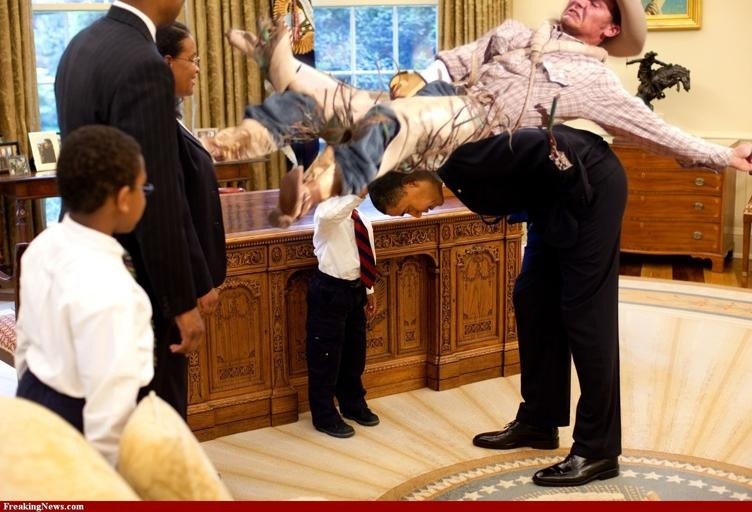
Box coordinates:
[371,446,752,501]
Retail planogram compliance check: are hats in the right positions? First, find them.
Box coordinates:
[598,0,646,56]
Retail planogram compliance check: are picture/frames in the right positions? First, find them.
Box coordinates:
[0,142,20,174]
[640,0,703,31]
[194,128,218,139]
[28,131,60,173]
[7,154,31,177]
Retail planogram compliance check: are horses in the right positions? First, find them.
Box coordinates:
[635,63,690,111]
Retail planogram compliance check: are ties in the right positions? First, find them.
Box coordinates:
[352,209,377,290]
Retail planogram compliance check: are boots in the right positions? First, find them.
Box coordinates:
[200,116,278,165]
[270,147,340,228]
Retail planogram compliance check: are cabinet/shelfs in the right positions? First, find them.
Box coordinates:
[609,139,741,273]
[188,187,525,443]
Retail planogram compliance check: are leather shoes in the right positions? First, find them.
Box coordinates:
[315,419,355,438]
[342,407,379,427]
[473,419,559,450]
[532,456,619,487]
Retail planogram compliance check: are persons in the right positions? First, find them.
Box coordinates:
[262,0,752,229]
[14,125,156,470]
[52,1,207,423]
[155,19,227,310]
[368,126,627,486]
[306,186,381,438]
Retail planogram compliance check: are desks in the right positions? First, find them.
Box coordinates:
[0,155,273,275]
